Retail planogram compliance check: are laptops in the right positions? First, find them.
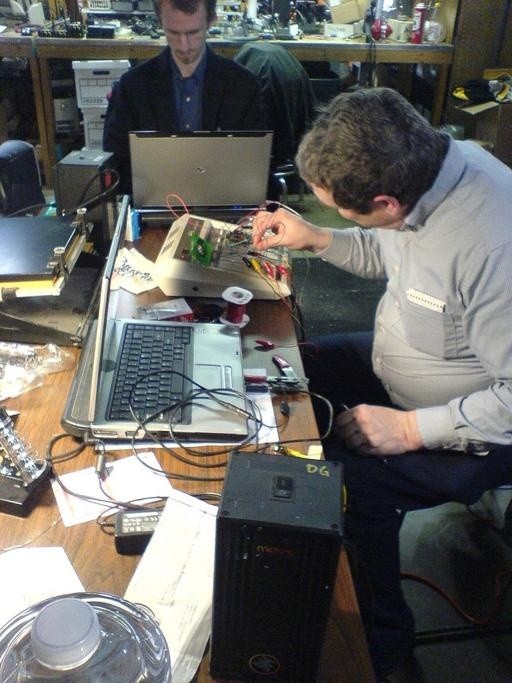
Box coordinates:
[89,193,247,442]
[128,128,275,209]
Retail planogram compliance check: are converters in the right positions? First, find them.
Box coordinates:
[114,508,164,549]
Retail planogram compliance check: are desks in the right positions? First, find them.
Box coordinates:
[0,206,379,682]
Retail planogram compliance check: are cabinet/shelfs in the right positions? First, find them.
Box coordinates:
[454,0,511,155]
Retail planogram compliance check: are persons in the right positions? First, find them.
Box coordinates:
[250,86,512,681]
[100,0,273,198]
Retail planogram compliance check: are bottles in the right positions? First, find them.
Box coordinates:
[410,2,427,44]
[427,3,443,43]
